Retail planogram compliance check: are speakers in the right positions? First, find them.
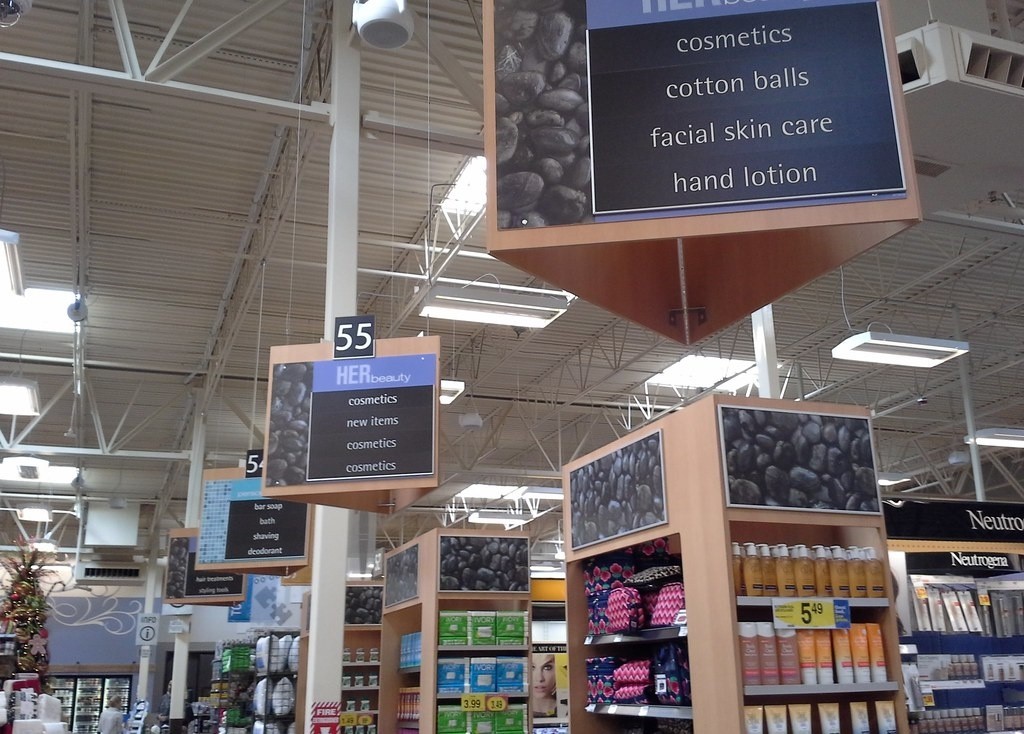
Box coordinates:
[353,0,414,49]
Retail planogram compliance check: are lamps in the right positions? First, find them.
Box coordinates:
[965,427,1024,448]
[0,229,28,297]
[27,538,58,553]
[513,486,565,500]
[16,502,53,523]
[419,286,568,329]
[468,511,533,526]
[832,331,968,370]
[0,375,42,417]
[440,379,465,405]
[878,472,910,486]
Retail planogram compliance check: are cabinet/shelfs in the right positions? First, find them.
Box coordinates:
[192,638,254,734]
[248,628,301,734]
[294,579,382,734]
[558,395,1024,734]
[378,528,569,734]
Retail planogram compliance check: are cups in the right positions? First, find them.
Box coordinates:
[343,648,379,734]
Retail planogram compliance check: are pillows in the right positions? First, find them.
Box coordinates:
[253,635,300,734]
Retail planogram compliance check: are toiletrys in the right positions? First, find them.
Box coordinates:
[727,534,901,734]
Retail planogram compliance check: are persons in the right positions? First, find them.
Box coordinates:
[159,680,172,716]
[532,654,558,727]
[98,696,129,734]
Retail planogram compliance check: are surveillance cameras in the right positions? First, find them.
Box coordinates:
[0,0,32,27]
